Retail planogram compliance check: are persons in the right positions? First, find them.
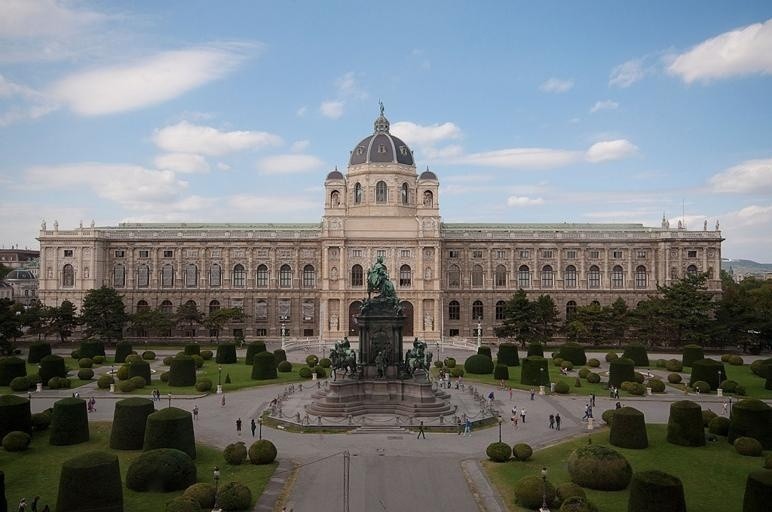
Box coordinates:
[18,496,50,512]
[695,386,700,399]
[220,395,226,408]
[511,406,528,431]
[251,419,256,437]
[417,421,425,439]
[72,392,80,398]
[282,508,293,512]
[582,394,596,421]
[88,397,96,413]
[192,404,199,421]
[151,387,160,402]
[723,401,728,414]
[608,385,619,399]
[329,256,433,382]
[486,380,535,401]
[550,413,561,431]
[236,418,241,435]
[438,369,463,389]
[457,416,471,439]
[560,366,568,377]
